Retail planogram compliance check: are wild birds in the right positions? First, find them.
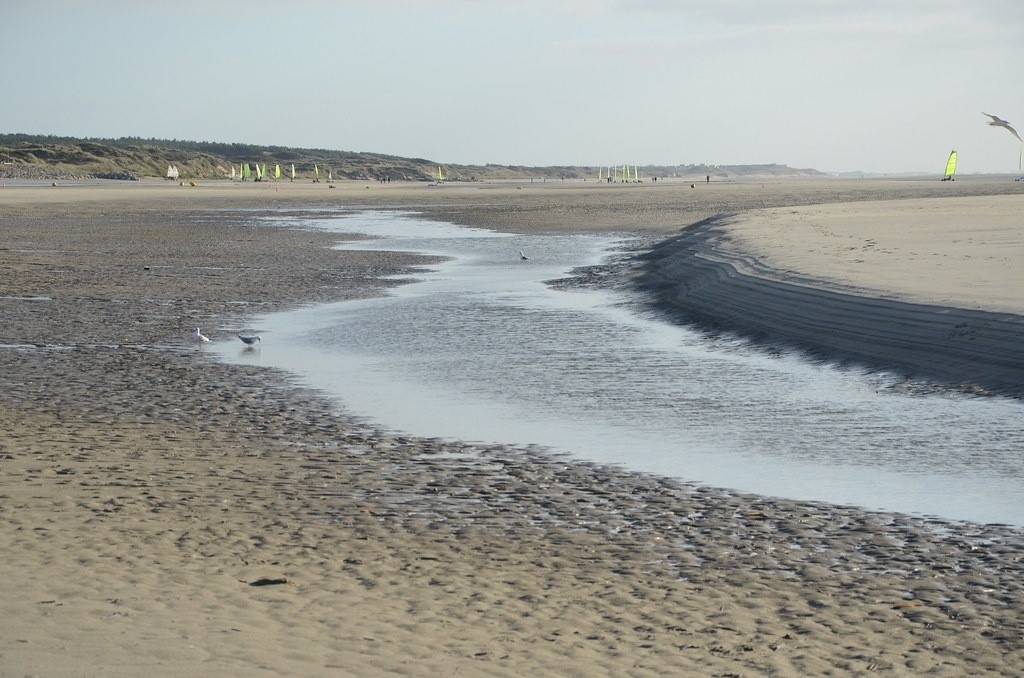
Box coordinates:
[238,335,261,350]
[519,251,531,261]
[194,327,210,350]
[981,112,1023,142]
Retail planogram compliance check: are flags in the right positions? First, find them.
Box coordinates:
[945,152,956,175]
[314,164,319,179]
[291,163,295,178]
[598,165,638,179]
[437,165,442,182]
[229,162,266,180]
[276,165,280,178]
[167,165,179,179]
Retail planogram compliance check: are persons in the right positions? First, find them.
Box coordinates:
[380,175,390,183]
[707,175,710,182]
